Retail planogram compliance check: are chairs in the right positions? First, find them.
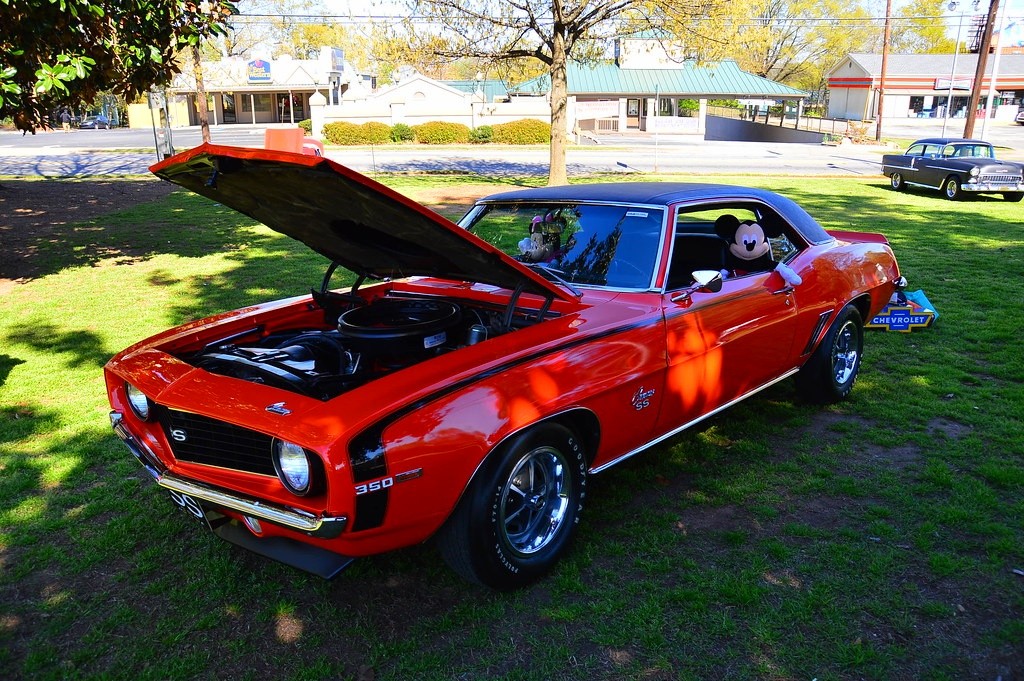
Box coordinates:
[672,233,731,284]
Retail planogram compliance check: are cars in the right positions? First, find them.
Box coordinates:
[79,115,110,130]
[74,112,129,129]
[881,137,1024,202]
[101,140,911,592]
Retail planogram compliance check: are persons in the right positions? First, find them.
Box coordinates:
[284,98,290,116]
[59,107,71,133]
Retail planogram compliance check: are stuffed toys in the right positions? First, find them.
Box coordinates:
[714,212,802,285]
[518,213,567,268]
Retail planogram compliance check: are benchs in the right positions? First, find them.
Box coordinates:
[917,109,935,117]
